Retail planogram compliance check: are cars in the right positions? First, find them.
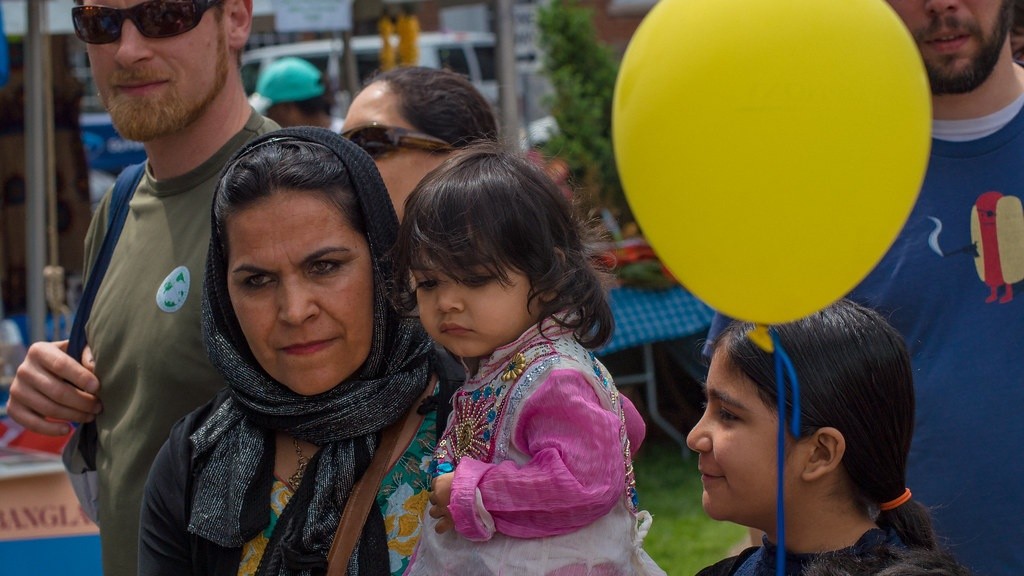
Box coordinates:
[241,31,523,121]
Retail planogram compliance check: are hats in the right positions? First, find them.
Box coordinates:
[247,57,325,112]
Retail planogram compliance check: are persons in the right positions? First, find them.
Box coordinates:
[844,2,1024,576]
[391,139,667,575]
[132,119,475,576]
[685,295,972,576]
[4,0,291,573]
[252,55,350,135]
[333,61,502,222]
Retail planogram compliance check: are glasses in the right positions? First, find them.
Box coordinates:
[71,0,213,46]
[340,124,455,158]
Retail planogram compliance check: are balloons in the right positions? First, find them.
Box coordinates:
[611,0,938,350]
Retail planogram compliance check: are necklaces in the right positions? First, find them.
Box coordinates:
[289,435,316,492]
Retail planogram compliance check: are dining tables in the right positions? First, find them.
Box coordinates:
[575,275,715,464]
